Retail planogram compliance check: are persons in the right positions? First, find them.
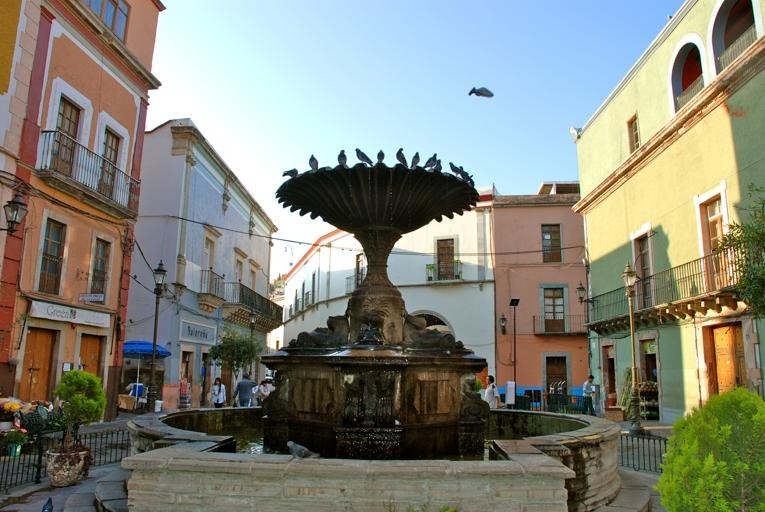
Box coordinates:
[582,375,598,415]
[211,377,227,409]
[483,375,505,409]
[232,373,270,406]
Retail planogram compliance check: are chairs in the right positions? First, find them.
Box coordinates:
[497,390,594,416]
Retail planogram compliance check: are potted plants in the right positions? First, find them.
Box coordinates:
[46,367,107,490]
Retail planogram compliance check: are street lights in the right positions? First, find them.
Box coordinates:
[620,259,642,436]
[248,308,257,379]
[147,259,168,412]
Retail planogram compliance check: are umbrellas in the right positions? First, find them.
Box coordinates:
[117,340,171,408]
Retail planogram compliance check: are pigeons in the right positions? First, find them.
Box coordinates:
[338,149,347,166]
[40,496,54,512]
[309,154,319,171]
[354,147,475,187]
[283,168,299,180]
[284,440,315,459]
